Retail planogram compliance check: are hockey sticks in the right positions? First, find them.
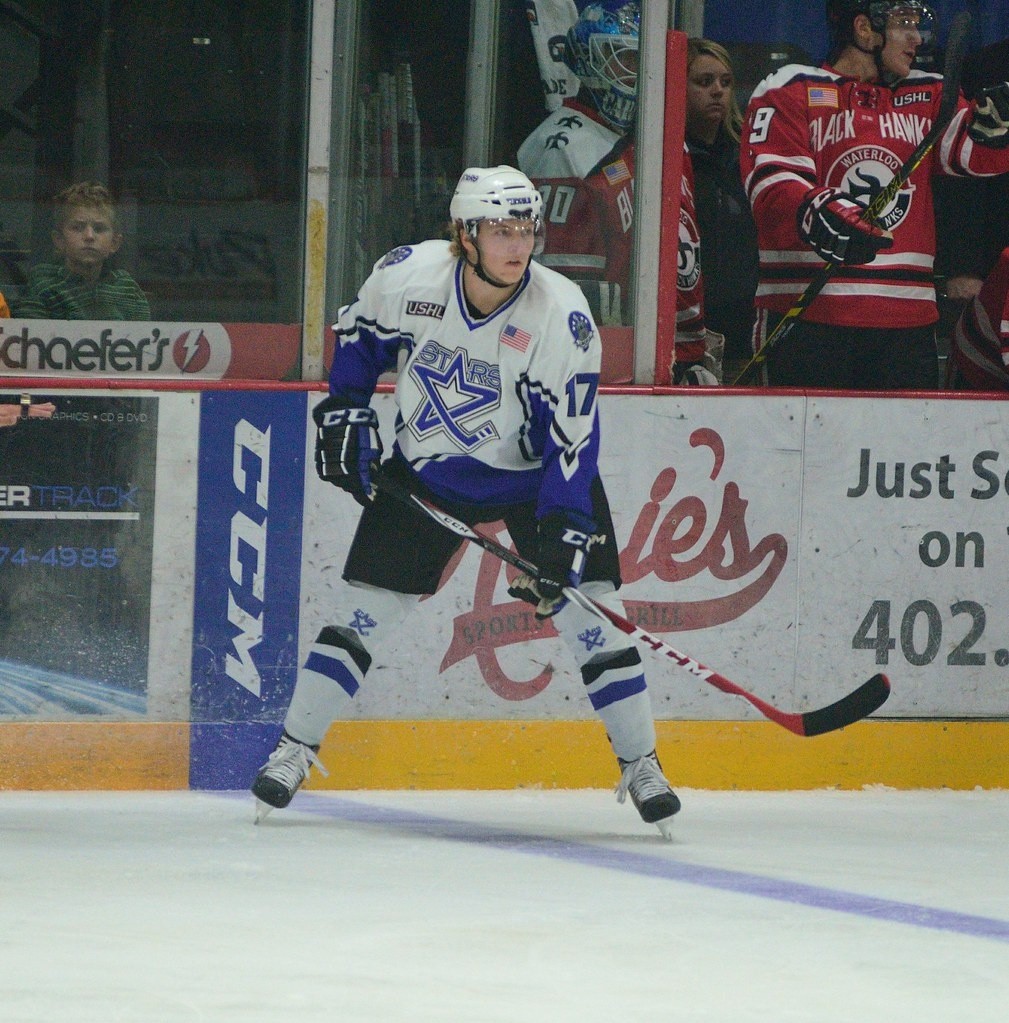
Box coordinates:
[727,5,975,387]
[369,471,894,740]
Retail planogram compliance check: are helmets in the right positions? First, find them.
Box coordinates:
[562,0,641,127]
[826,0,924,32]
[449,164,544,241]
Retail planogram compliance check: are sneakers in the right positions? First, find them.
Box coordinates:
[250,726,328,826]
[612,749,680,839]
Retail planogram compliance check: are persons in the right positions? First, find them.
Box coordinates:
[740,0,1009,392]
[941,249,1009,392]
[515,0,723,389]
[10,181,152,636]
[683,38,760,383]
[250,165,681,838]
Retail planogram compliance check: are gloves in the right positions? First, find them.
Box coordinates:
[967,81,1009,148]
[509,506,596,620]
[313,404,385,500]
[794,186,893,264]
